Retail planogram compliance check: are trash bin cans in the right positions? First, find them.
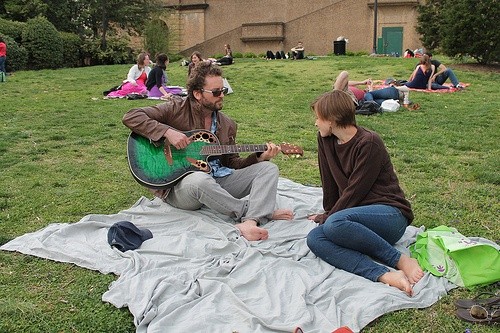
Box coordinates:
[333,38,346,56]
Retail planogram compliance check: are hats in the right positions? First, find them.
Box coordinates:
[107,220,154,253]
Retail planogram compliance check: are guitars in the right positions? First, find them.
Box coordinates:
[127,121,305,190]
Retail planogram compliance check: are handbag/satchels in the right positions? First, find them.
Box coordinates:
[354,99,401,115]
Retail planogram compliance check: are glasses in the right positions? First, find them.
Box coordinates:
[201,87,228,97]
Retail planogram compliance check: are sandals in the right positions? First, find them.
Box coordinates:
[454,292,500,322]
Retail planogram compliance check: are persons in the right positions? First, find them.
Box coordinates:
[0,37,7,82]
[333,47,465,109]
[188,51,203,75]
[147,53,182,99]
[122,62,293,240]
[266,41,305,60]
[307,91,424,298]
[106,54,152,98]
[214,43,233,66]
